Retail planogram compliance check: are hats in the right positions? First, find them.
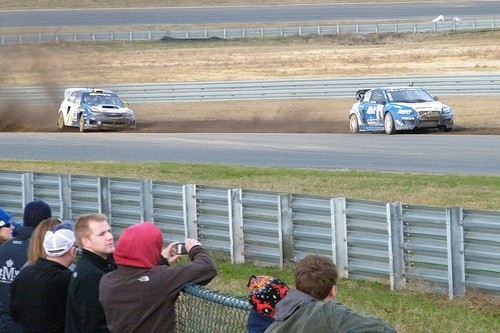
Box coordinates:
[0,208,14,227]
[42,220,77,256]
[247,275,290,318]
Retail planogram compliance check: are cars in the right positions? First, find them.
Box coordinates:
[349,85,456,135]
[56,86,135,132]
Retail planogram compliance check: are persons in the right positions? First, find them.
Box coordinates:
[245,274,291,333]
[0,206,24,252]
[102,221,217,333]
[264,254,399,333]
[15,216,77,282]
[0,200,53,333]
[63,213,118,333]
[11,219,77,333]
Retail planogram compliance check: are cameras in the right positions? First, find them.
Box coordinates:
[176,243,188,254]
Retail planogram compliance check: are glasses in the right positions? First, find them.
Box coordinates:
[0,223,10,228]
